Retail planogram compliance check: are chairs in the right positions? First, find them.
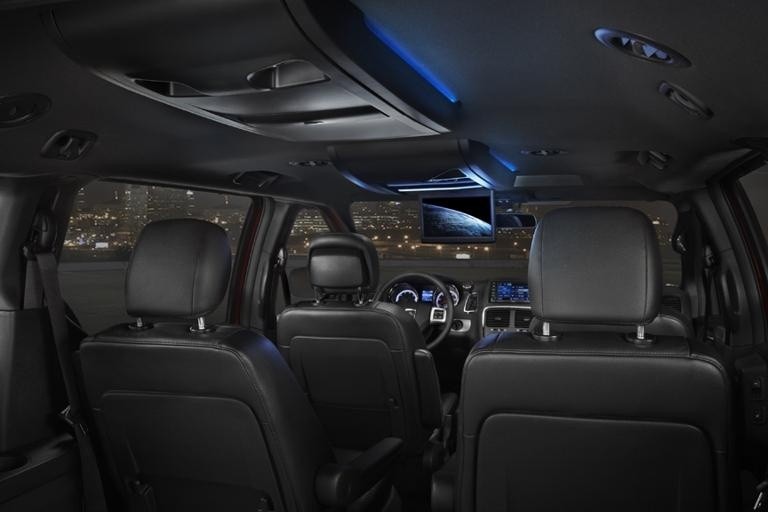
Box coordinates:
[80,215,413,512]
[277,232,457,512]
